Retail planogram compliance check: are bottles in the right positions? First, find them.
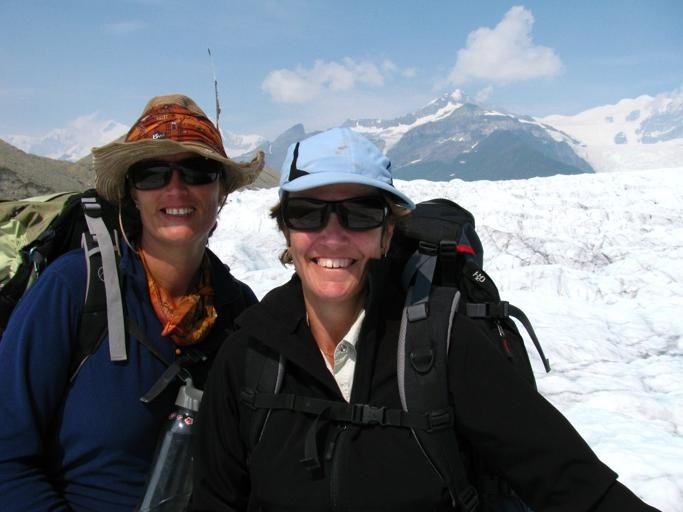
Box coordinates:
[135,376,204,512]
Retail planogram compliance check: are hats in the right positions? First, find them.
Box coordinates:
[278,127,418,210]
[92,93,266,204]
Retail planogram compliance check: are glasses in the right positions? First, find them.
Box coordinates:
[126,153,226,189]
[274,195,396,230]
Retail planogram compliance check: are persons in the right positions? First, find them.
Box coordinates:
[193,127,660,512]
[0,95,265,512]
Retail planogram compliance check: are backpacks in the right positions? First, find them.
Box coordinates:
[0,187,128,387]
[371,198,550,512]
